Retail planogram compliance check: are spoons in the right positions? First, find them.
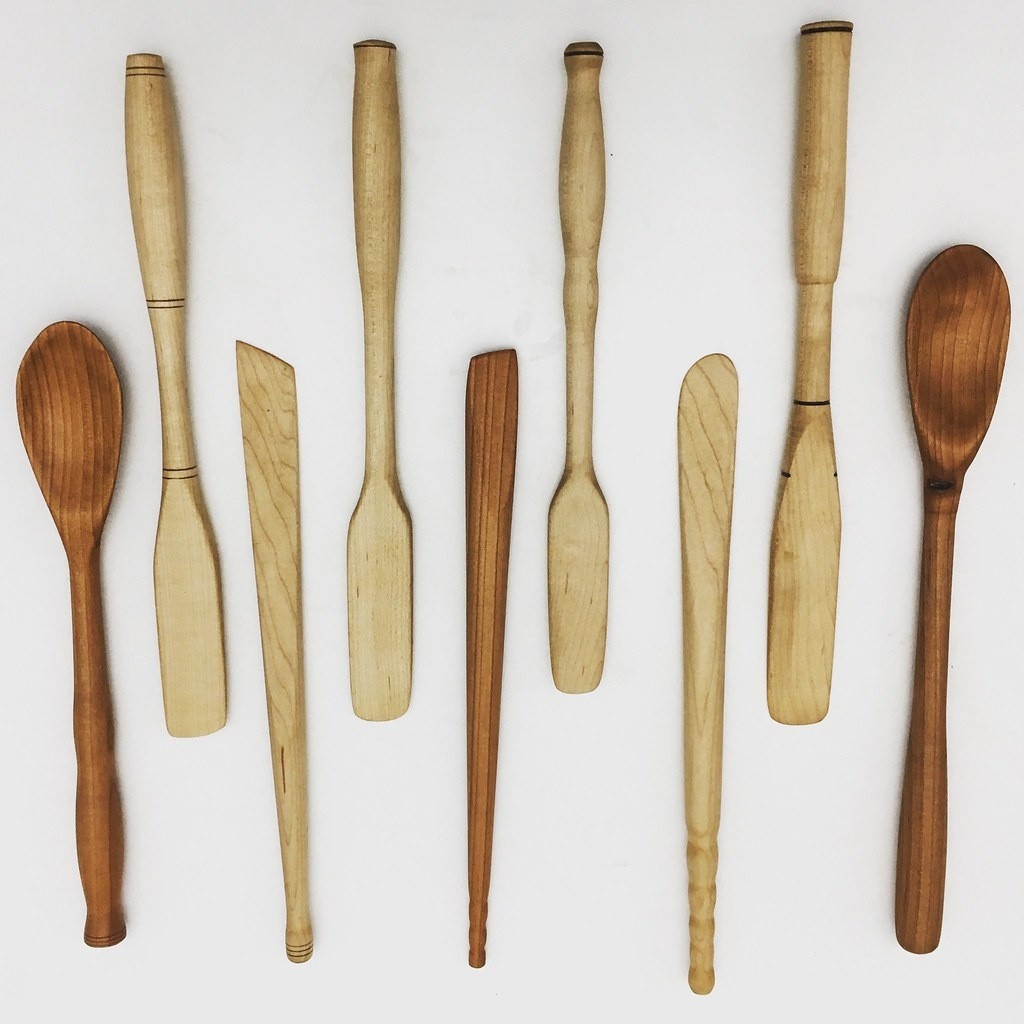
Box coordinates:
[12,321,129,952]
[891,242,1010,956]
